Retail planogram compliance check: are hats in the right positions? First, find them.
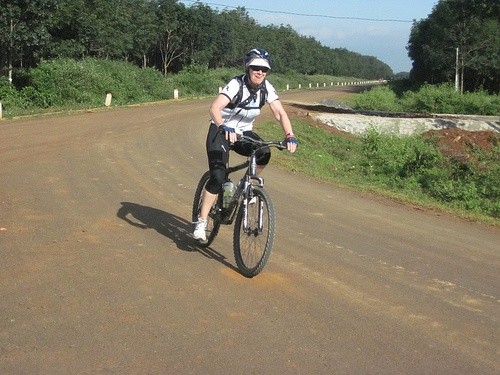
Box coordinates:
[245,49,273,70]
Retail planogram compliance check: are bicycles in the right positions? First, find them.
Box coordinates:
[190,131,291,277]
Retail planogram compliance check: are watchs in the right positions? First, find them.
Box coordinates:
[286,131,294,136]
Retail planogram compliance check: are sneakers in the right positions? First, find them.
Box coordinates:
[193,218,207,241]
[238,180,256,205]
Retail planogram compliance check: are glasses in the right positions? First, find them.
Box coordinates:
[249,66,269,72]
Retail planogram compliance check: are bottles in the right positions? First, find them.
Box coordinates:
[222,179,235,209]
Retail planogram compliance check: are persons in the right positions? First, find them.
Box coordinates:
[193,47,299,242]
